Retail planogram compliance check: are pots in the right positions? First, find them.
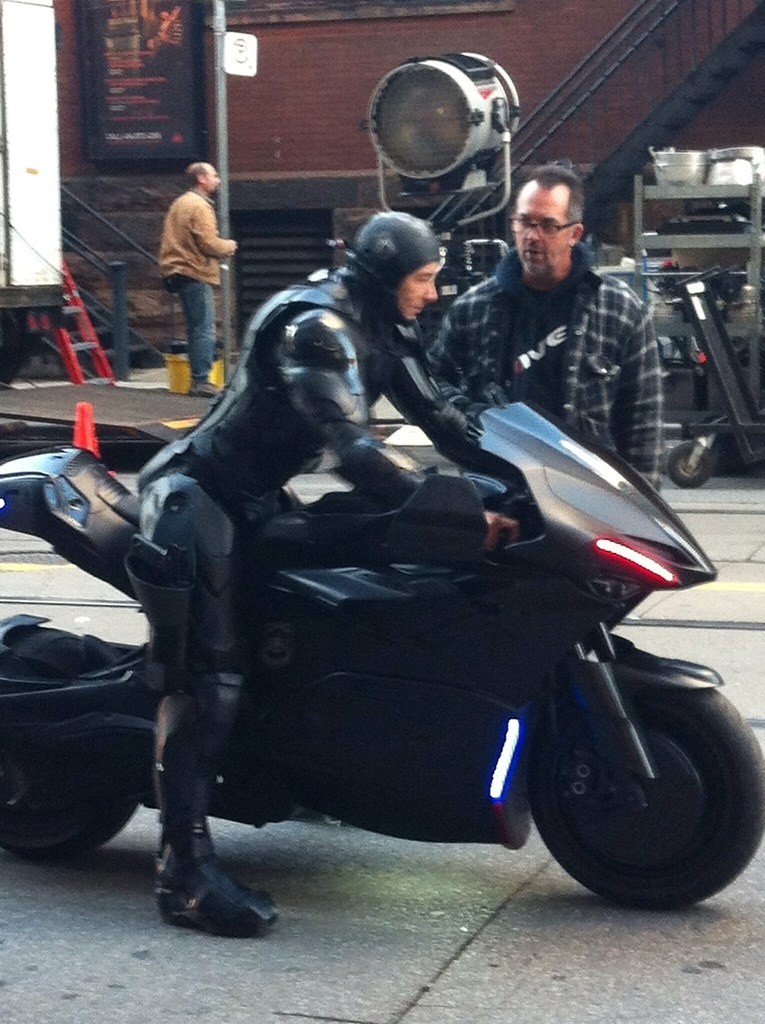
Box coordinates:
[649,142,708,186]
[708,145,765,187]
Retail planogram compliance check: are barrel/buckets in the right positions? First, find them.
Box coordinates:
[161,352,225,396]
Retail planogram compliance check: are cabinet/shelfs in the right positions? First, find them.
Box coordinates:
[630,174,765,423]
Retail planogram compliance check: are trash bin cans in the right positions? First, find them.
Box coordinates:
[160,351,224,394]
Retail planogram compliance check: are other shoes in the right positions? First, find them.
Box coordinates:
[188,381,217,398]
[154,860,276,941]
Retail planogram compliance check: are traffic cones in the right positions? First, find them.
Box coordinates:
[71,399,120,479]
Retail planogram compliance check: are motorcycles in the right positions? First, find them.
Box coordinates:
[0,393,765,909]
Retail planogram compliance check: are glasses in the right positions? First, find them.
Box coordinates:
[507,212,585,241]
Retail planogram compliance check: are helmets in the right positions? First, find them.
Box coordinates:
[342,211,440,327]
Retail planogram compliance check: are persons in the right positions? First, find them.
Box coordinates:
[125,211,522,938]
[424,164,663,496]
[156,162,238,398]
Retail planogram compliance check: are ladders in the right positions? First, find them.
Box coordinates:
[49,261,116,384]
